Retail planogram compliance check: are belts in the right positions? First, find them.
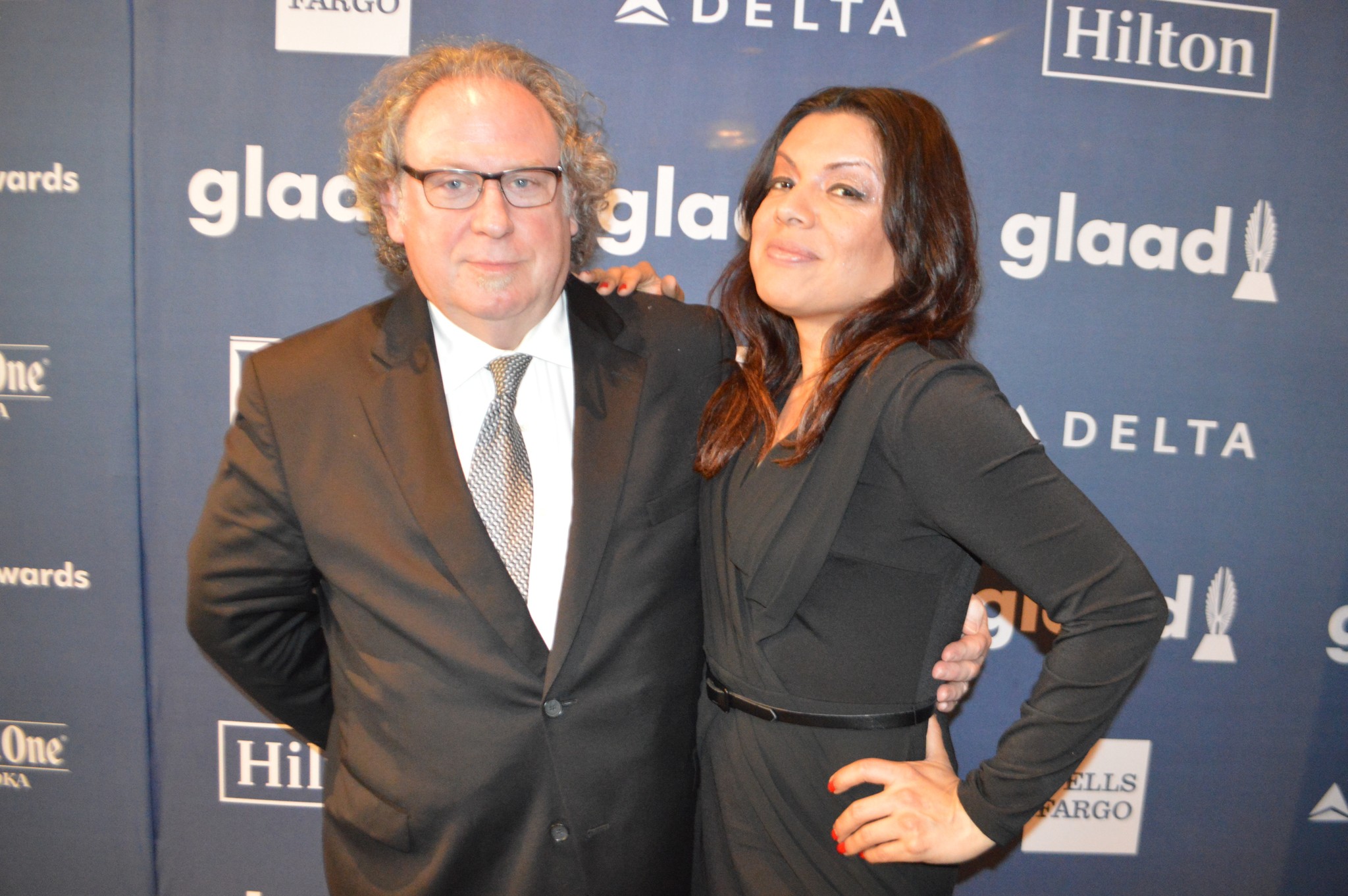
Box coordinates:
[703,672,938,732]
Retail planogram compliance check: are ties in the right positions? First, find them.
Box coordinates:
[467,353,532,604]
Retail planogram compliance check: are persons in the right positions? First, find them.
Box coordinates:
[185,50,990,896]
[565,87,1168,895]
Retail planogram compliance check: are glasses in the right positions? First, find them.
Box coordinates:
[395,158,562,211]
